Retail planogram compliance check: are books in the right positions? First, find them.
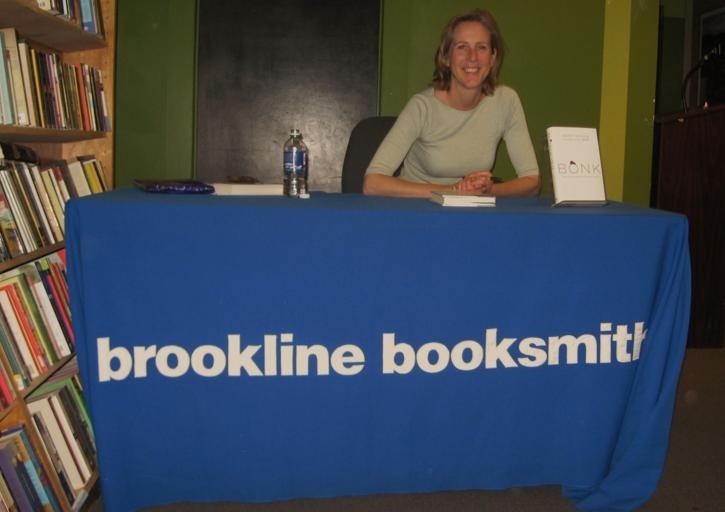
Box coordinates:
[0,248,98,511]
[429,189,496,207]
[1,141,111,263]
[2,0,110,134]
[546,125,606,204]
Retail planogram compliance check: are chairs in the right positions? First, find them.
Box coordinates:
[340,115,406,197]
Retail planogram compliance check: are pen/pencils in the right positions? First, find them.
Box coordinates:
[461,176,505,181]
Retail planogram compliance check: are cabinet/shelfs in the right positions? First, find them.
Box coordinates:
[1,0,117,511]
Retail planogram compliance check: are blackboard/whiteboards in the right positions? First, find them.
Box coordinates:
[191,1,385,193]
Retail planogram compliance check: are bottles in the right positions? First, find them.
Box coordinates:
[283,129,307,199]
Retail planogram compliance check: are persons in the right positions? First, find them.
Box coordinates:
[361,7,542,198]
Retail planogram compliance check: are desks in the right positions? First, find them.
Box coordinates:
[63,187,689,510]
